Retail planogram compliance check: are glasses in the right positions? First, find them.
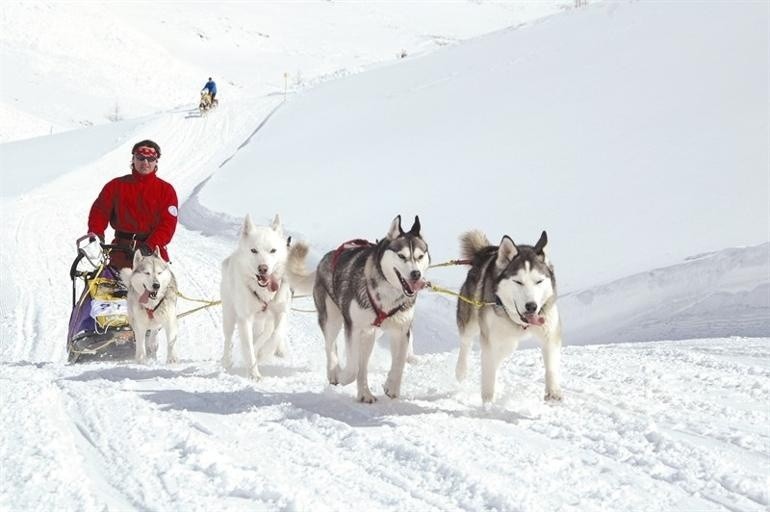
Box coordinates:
[135,153,157,162]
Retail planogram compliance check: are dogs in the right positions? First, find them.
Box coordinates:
[119,245,178,365]
[199,100,218,118]
[219,212,292,380]
[284,214,430,402]
[457,227,566,403]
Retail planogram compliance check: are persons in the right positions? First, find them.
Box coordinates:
[201,77,217,103]
[88,140,178,269]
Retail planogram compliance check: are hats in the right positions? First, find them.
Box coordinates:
[132,140,161,159]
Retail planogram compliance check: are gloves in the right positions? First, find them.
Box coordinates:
[140,243,152,256]
